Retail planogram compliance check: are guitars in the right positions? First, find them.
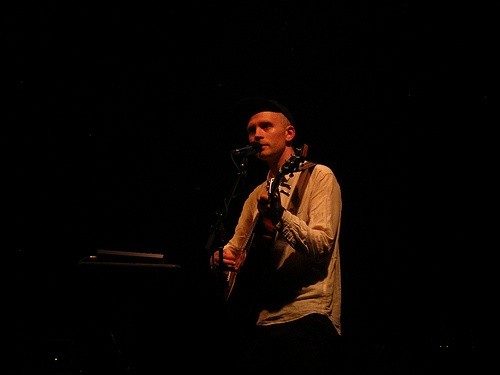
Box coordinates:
[223,143,309,304]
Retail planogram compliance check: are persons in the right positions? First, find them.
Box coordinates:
[210,100,343,375]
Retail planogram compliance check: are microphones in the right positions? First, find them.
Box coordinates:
[231,141,261,156]
